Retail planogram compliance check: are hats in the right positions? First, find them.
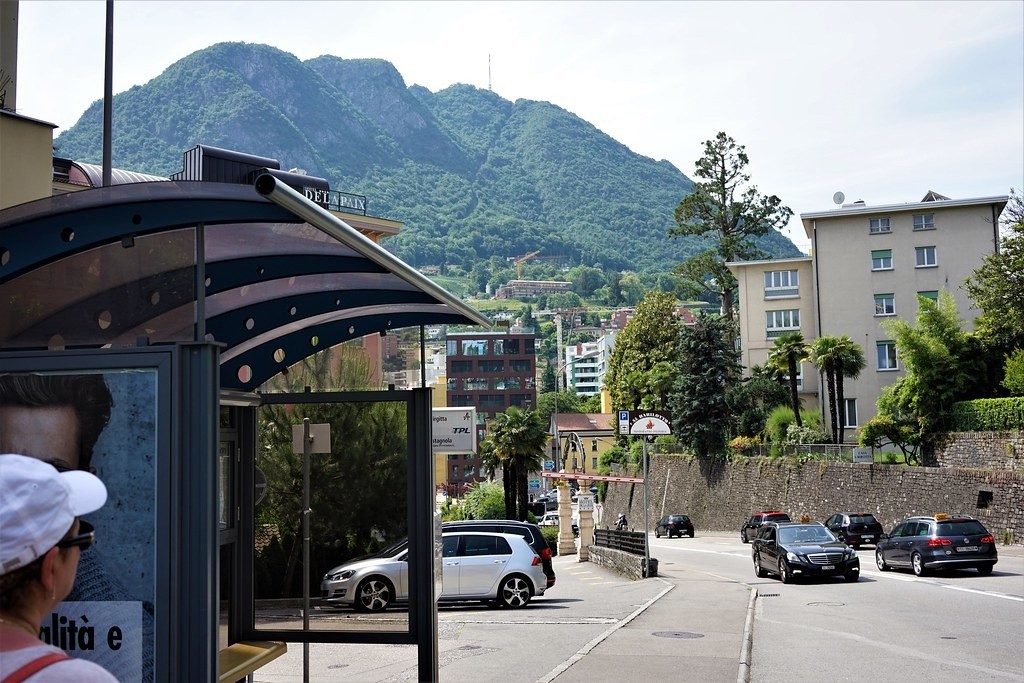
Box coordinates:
[1,454,107,576]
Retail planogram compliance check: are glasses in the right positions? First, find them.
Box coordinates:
[55,521,96,552]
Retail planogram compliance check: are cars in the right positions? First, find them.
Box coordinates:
[751,516,860,585]
[535,487,598,525]
[823,511,884,548]
[320,531,547,613]
[875,513,998,576]
[654,514,695,538]
[741,511,791,543]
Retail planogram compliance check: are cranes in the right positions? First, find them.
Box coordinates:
[516,247,545,280]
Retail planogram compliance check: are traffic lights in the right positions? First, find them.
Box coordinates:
[530,494,533,502]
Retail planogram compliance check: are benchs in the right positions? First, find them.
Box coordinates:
[217,639,289,683]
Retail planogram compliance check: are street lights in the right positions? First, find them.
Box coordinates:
[555,349,600,473]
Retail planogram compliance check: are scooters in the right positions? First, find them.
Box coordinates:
[614,523,628,531]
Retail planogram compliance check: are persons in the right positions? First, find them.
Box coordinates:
[0,374,154,683]
[614,515,628,530]
[0,454,120,683]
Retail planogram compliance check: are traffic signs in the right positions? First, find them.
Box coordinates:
[529,478,541,489]
[545,460,554,470]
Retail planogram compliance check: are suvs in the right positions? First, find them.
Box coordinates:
[345,519,556,606]
